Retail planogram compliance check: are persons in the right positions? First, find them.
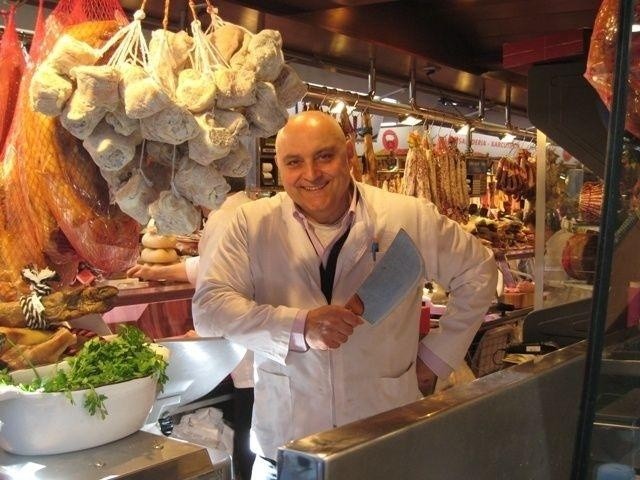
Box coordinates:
[125,175,257,479]
[467,201,574,310]
[191,110,498,479]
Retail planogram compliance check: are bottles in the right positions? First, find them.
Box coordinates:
[420,301,430,333]
[626,281,639,329]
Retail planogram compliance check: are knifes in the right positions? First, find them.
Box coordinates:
[344,227,425,328]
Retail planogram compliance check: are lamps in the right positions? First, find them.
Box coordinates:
[454,123,470,137]
[326,98,344,114]
[398,113,423,126]
[498,133,515,143]
